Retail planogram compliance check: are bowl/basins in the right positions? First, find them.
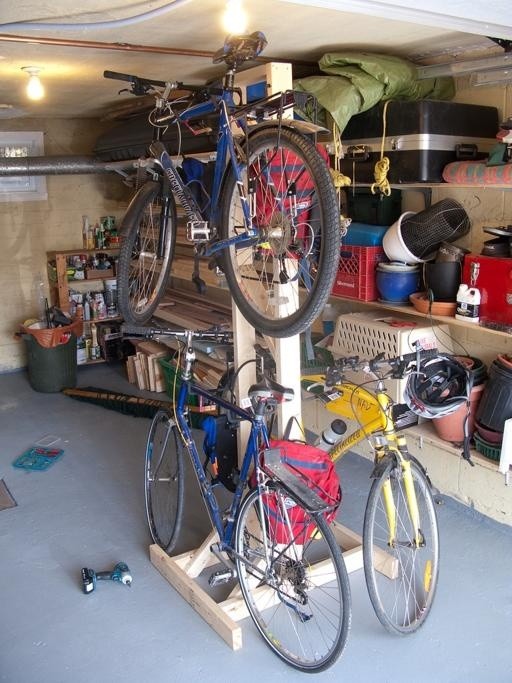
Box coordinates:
[375,267,420,301]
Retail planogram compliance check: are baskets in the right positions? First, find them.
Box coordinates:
[311,245,385,304]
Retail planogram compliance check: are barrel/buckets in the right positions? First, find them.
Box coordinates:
[456,282,483,324]
[76,336,88,366]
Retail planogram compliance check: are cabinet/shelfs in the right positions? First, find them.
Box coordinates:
[46,182,512,527]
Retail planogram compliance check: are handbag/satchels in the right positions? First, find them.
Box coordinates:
[249,439,341,546]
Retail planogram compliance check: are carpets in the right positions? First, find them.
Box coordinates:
[0,478,18,511]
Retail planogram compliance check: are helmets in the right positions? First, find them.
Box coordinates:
[403,352,474,419]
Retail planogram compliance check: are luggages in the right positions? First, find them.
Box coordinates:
[335,98,505,186]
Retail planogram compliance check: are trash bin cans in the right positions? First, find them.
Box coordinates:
[22,317,79,394]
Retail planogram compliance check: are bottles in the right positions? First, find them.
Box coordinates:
[69,289,107,319]
[73,255,111,279]
[90,325,102,361]
[82,215,107,251]
[312,419,347,454]
[323,303,336,335]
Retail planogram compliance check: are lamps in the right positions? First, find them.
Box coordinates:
[20,66,44,101]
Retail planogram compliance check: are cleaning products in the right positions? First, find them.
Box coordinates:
[82,215,90,249]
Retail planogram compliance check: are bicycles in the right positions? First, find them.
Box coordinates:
[201,338,476,638]
[120,324,351,674]
[102,30,342,339]
